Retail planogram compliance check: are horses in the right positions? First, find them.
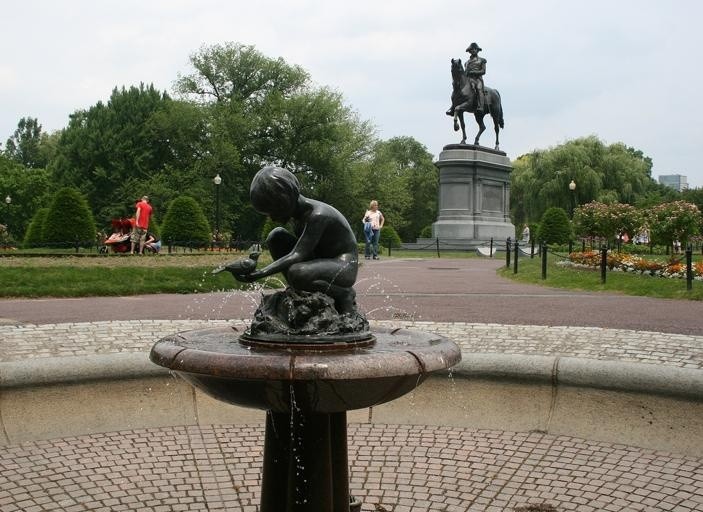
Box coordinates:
[451,57,504,151]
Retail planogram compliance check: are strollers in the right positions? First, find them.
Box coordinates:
[104,217,140,254]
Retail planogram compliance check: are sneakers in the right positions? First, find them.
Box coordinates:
[365,255,380,260]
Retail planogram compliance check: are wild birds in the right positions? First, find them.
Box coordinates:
[211,252,261,275]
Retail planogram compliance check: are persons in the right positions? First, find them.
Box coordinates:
[362,199,386,260]
[224,162,362,319]
[464,40,487,114]
[129,195,153,257]
[137,230,162,255]
[521,223,531,246]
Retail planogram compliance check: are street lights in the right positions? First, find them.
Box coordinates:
[5,195,12,236]
[214,174,222,234]
[569,180,577,221]
